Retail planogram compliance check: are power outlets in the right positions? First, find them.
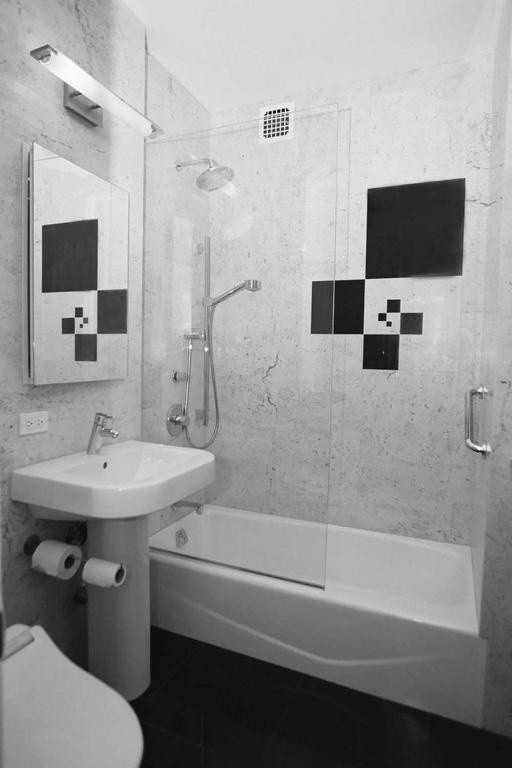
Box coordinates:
[18,411,49,437]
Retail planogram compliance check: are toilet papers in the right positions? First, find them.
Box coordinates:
[30,538,82,582]
[81,557,127,588]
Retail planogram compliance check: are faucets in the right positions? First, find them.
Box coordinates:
[86,411,119,455]
[171,500,204,516]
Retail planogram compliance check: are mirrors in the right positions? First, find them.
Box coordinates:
[29,143,132,386]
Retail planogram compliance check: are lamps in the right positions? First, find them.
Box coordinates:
[31,43,165,139]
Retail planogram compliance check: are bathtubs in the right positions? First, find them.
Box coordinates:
[149,501,487,728]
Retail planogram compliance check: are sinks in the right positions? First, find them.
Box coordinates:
[11,439,216,522]
